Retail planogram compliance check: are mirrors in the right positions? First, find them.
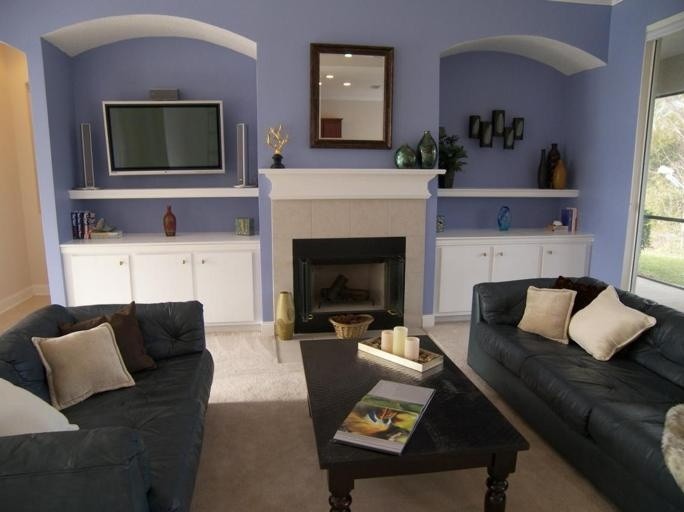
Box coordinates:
[309,41,395,150]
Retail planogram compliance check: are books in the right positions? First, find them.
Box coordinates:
[72,209,122,239]
[329,380,436,456]
[548,208,577,232]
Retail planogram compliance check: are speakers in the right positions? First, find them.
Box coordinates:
[71,121,105,190]
[233,121,257,188]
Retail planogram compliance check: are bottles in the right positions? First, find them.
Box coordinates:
[163,205,177,237]
[538,141,568,190]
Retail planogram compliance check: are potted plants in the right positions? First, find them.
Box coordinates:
[438,122,467,188]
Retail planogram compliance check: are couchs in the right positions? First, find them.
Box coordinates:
[0,300,215,512]
[463,274,684,512]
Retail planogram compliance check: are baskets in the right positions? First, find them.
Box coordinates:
[329,314,374,339]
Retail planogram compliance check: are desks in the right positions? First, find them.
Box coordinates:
[298,328,531,512]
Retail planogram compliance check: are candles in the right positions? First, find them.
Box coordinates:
[379,329,392,352]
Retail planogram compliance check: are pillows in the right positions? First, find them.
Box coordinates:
[0,376,79,440]
[29,322,138,415]
[517,283,578,345]
[567,281,656,362]
[552,275,608,346]
[66,303,161,373]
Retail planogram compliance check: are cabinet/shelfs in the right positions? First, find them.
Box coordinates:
[435,227,539,324]
[320,116,343,139]
[535,223,595,282]
[131,230,264,334]
[61,229,133,327]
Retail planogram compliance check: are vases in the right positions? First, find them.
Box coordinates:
[495,205,512,233]
[162,202,176,238]
[395,141,417,169]
[546,141,560,186]
[535,149,551,188]
[416,128,437,169]
[553,160,567,191]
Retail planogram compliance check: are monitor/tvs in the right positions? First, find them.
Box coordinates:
[101,99,226,175]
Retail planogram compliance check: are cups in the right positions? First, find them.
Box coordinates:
[380,329,395,352]
